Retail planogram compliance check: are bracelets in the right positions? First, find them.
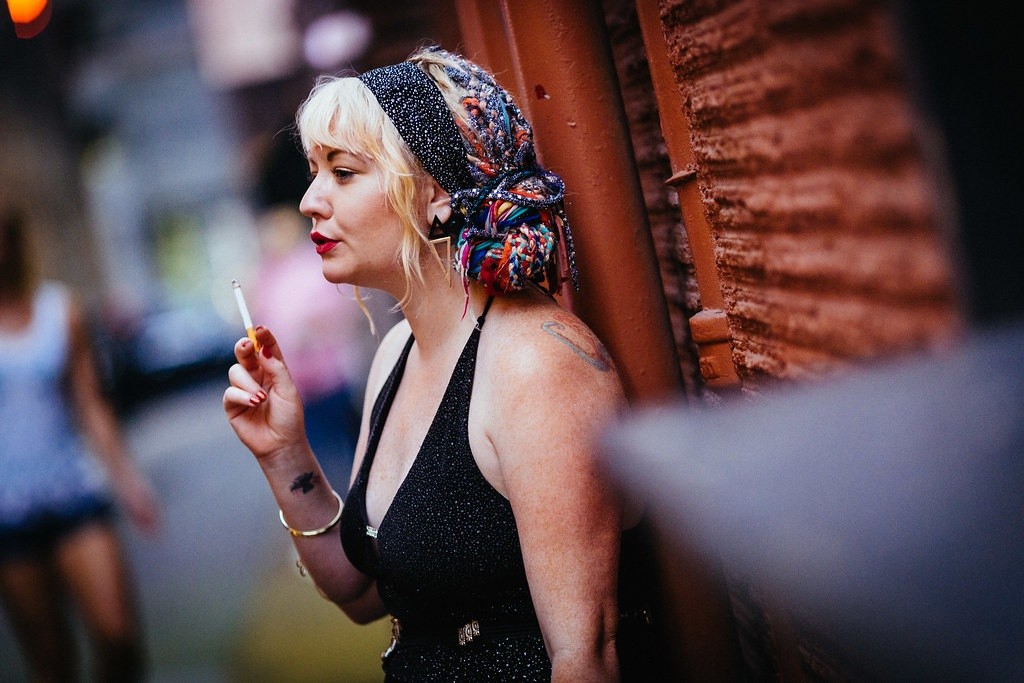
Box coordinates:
[279,489,344,536]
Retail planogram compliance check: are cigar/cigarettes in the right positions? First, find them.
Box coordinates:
[231,279,260,354]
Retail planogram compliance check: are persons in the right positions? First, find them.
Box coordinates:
[0,206,158,683]
[223,46,632,683]
